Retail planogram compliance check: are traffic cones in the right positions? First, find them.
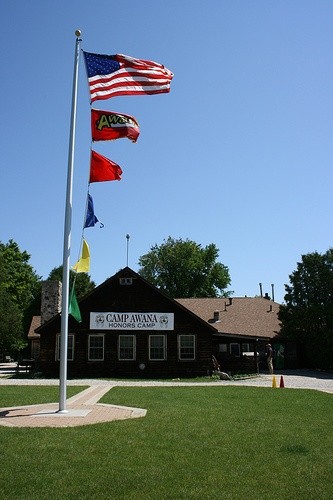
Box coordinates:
[271,375,277,389]
[279,375,285,388]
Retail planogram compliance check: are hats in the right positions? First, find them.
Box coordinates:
[266,343,271,346]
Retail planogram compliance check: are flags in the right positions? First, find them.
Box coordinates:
[84,51,173,101]
[73,240,90,273]
[69,287,82,323]
[91,108,140,142]
[83,194,104,228]
[90,151,122,183]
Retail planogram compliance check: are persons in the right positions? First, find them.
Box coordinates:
[266,344,274,374]
